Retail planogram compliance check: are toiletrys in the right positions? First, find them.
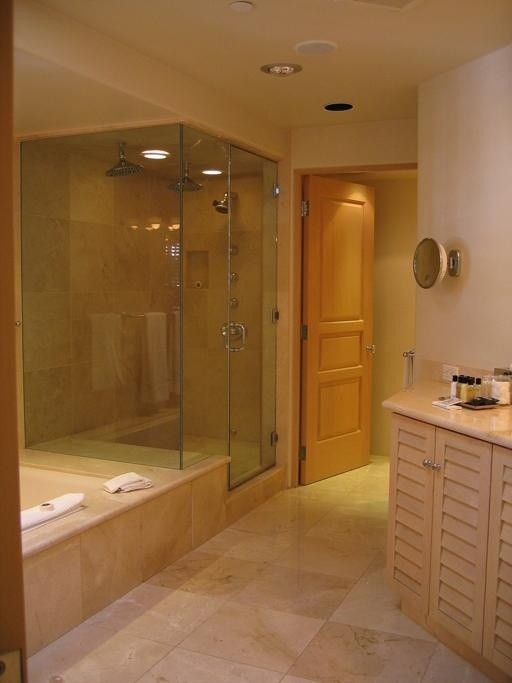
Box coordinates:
[450,374,483,402]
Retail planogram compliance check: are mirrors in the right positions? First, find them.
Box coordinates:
[410,236,460,288]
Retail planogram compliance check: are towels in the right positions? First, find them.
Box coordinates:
[20,470,155,534]
[402,347,414,389]
[89,310,187,406]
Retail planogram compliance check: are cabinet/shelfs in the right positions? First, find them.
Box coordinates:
[388,412,512,683]
